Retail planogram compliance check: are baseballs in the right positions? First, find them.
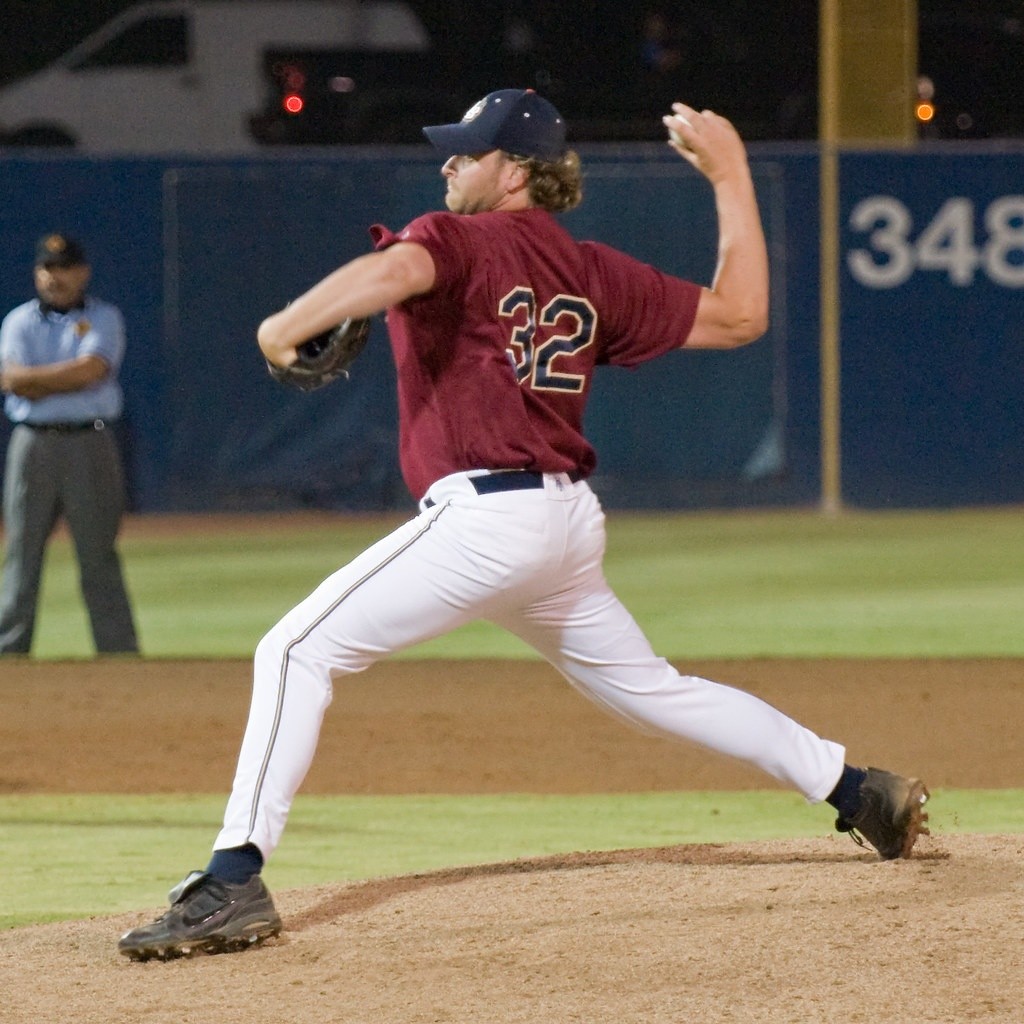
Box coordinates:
[668,112,693,148]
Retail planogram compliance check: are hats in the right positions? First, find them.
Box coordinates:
[421,88,567,163]
[34,232,81,270]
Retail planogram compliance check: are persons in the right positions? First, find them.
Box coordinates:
[117,89,933,966]
[0,235,138,658]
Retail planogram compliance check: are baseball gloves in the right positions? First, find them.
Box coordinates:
[263,297,373,384]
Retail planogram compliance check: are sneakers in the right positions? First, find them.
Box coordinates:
[117,869,283,963]
[834,764,931,860]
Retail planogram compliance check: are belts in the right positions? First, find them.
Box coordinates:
[16,421,111,435]
[423,467,580,506]
